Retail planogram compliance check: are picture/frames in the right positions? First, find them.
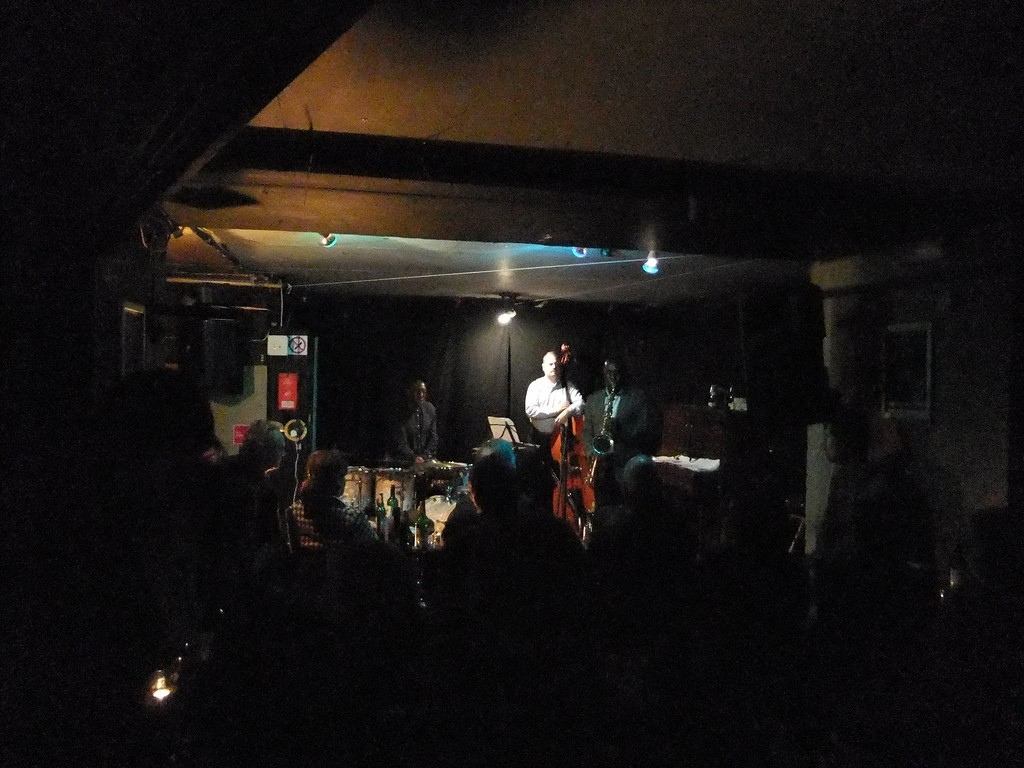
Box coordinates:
[877,320,935,419]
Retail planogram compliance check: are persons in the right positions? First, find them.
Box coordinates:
[524,350,586,442]
[581,360,648,507]
[442,384,729,554]
[395,380,438,464]
[228,419,373,551]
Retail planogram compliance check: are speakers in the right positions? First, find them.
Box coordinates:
[224,304,271,366]
[176,315,240,395]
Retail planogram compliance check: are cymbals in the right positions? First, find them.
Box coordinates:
[413,456,468,468]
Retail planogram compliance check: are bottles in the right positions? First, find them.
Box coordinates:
[389,509,400,548]
[386,485,398,520]
[414,502,429,553]
[708,384,718,409]
[376,493,386,540]
[364,488,375,530]
[728,387,735,411]
[432,532,440,550]
[400,516,413,554]
[949,553,961,592]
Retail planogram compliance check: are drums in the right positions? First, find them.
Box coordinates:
[339,465,373,504]
[373,467,416,518]
[412,495,459,549]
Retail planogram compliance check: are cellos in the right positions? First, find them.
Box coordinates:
[549,339,597,548]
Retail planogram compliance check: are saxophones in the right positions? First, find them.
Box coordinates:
[591,381,617,460]
